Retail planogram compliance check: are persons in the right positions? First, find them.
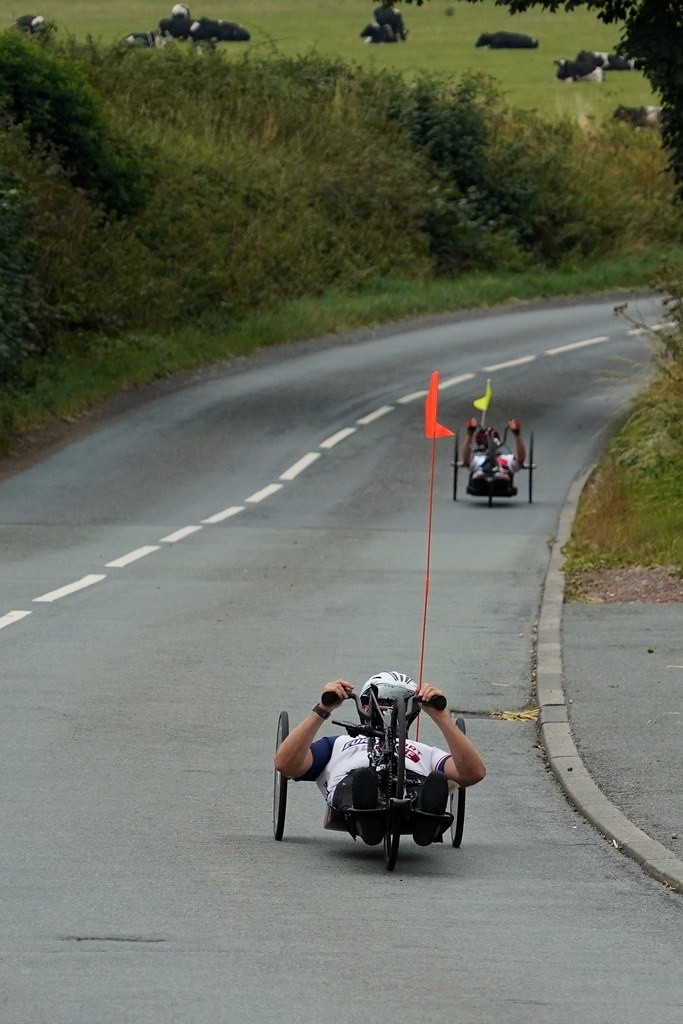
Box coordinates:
[461,417,525,496]
[274,671,486,846]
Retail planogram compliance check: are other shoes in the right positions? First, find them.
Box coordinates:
[352,767,388,848]
[413,772,449,847]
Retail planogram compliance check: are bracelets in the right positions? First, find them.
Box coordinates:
[312,703,331,720]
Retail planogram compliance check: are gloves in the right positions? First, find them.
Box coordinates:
[466,417,478,435]
[508,418,521,436]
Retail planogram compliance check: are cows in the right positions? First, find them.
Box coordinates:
[610,104,670,130]
[360,7,410,44]
[553,50,636,83]
[121,3,250,48]
[476,30,539,49]
[14,15,46,33]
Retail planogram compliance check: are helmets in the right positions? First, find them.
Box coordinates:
[475,425,501,449]
[359,671,420,731]
